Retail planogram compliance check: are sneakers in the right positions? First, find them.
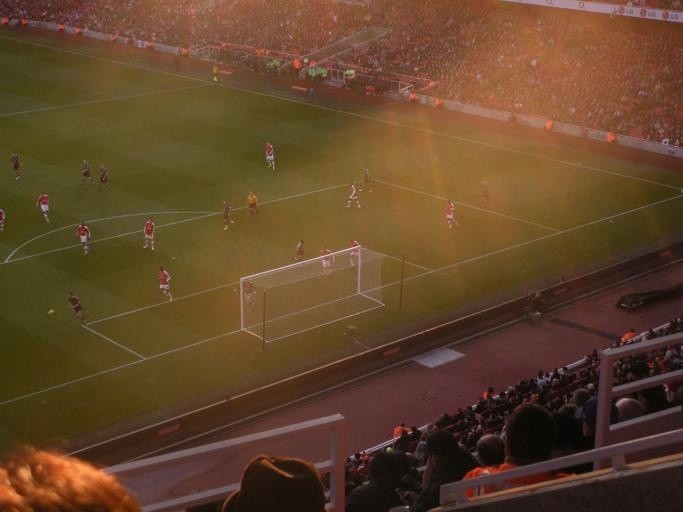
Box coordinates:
[166,296,172,303]
[83,247,90,256]
[143,244,154,250]
[268,165,275,170]
[224,221,235,230]
[345,204,361,208]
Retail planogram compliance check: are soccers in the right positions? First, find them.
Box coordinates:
[47,309,55,316]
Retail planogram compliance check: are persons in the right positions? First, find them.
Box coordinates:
[264,141,275,171]
[154,266,172,303]
[218,164,374,279]
[441,199,458,229]
[0,152,110,256]
[68,292,86,326]
[142,217,155,250]
[0,442,143,512]
[0,0,683,147]
[310,317,683,512]
[233,277,257,312]
[479,180,493,210]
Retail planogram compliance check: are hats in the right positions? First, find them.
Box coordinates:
[504,402,561,445]
[559,389,643,438]
[426,431,453,455]
